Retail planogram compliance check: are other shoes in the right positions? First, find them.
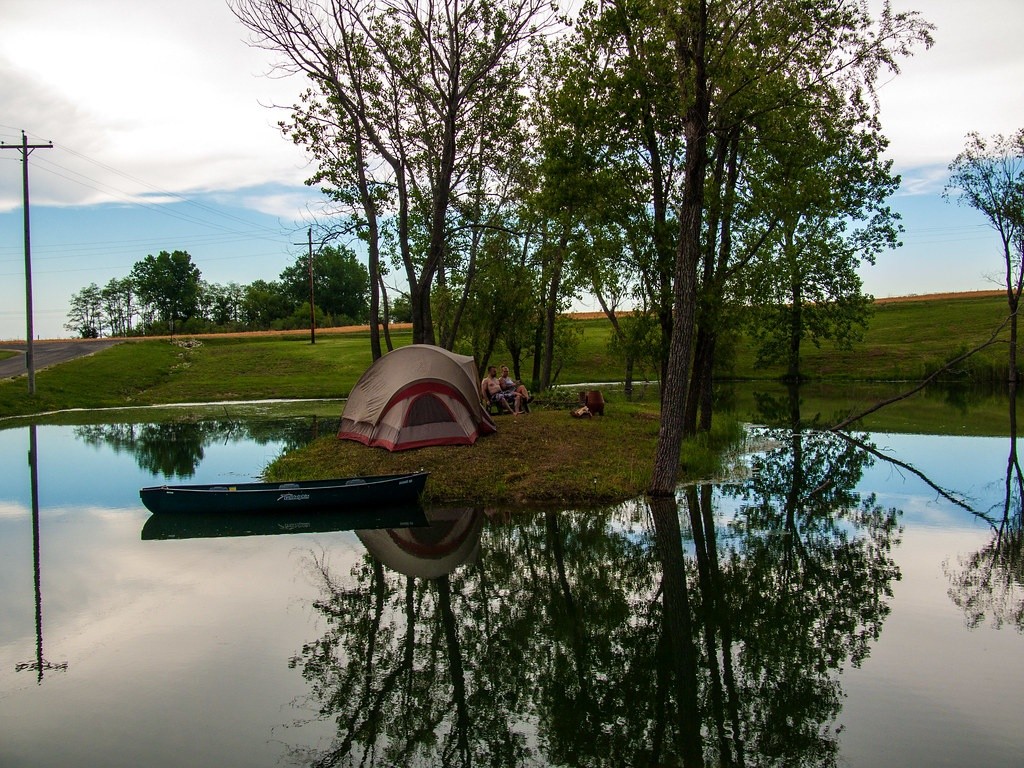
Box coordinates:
[528,396,534,403]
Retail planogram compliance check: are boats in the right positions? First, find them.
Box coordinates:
[140,499,431,541]
[140,468,435,517]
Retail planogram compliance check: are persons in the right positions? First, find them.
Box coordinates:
[481,365,535,416]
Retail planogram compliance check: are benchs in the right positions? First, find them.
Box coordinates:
[485,391,531,416]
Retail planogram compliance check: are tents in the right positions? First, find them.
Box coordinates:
[354,499,486,581]
[336,344,498,452]
